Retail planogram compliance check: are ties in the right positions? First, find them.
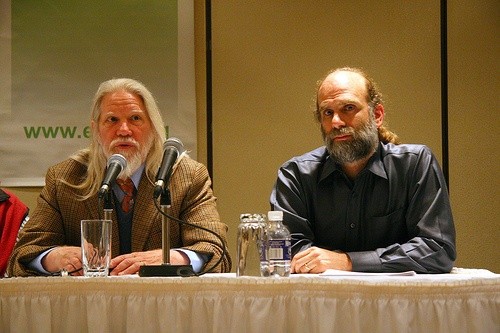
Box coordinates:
[117,176,138,214]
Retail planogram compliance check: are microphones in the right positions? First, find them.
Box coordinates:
[153,137,183,198]
[97,154,128,199]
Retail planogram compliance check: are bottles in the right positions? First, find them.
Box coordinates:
[260,210,291,277]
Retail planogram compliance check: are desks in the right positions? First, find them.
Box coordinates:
[0,268,500,333]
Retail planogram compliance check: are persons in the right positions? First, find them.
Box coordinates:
[0,188,30,278]
[270,68,457,274]
[8,79,232,275]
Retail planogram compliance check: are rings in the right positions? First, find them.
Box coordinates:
[304,263,310,270]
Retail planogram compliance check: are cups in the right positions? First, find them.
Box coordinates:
[235,213,266,277]
[80,219,112,277]
